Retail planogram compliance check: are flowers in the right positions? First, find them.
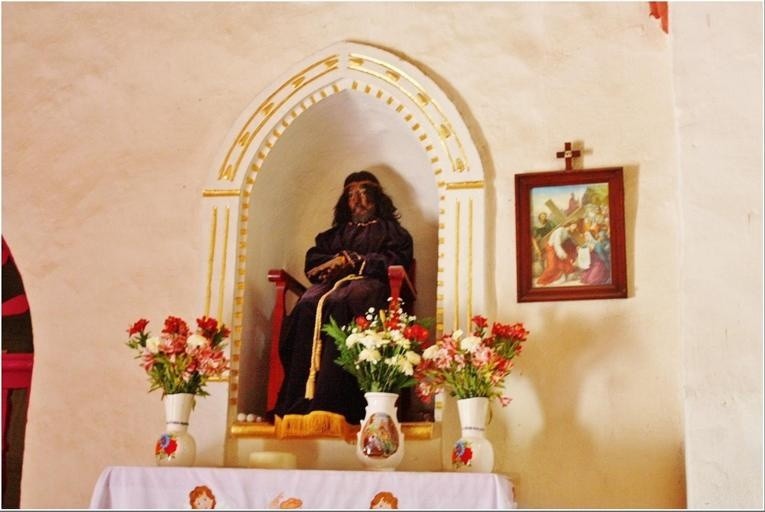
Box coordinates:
[320,295,435,394]
[120,315,236,406]
[401,303,531,404]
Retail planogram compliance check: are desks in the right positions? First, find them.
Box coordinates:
[84,460,519,511]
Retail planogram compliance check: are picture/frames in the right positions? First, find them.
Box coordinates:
[514,168,630,303]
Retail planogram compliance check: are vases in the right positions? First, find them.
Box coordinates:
[353,386,407,468]
[449,394,497,473]
[150,389,197,467]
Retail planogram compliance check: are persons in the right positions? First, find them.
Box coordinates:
[531,191,611,287]
[262,169,415,424]
